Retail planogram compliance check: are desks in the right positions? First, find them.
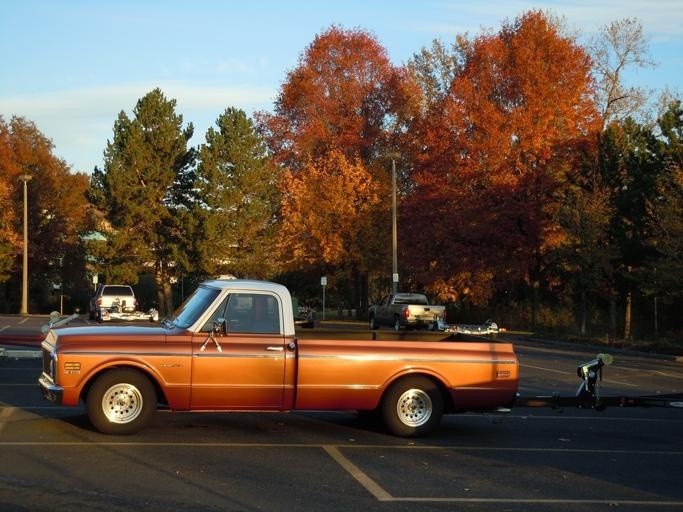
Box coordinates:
[19,175,35,314]
[383,150,402,295]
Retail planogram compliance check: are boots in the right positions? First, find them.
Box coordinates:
[89,283,139,323]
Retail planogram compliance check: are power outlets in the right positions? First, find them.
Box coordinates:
[366,291,447,335]
[37,279,520,435]
